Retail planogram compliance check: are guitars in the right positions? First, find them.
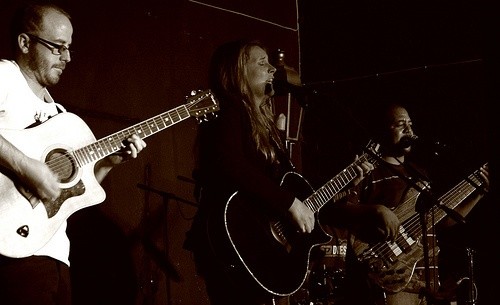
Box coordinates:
[349,161,496,294]
[0,88,222,258]
[224,138,387,297]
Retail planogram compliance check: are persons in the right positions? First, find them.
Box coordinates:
[0,0,147,305]
[338,99,493,305]
[183,40,375,305]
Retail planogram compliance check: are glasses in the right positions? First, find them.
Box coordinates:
[23,32,76,56]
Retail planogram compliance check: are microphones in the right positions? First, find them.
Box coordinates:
[408,135,439,144]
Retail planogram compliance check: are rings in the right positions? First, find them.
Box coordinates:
[365,171,372,175]
